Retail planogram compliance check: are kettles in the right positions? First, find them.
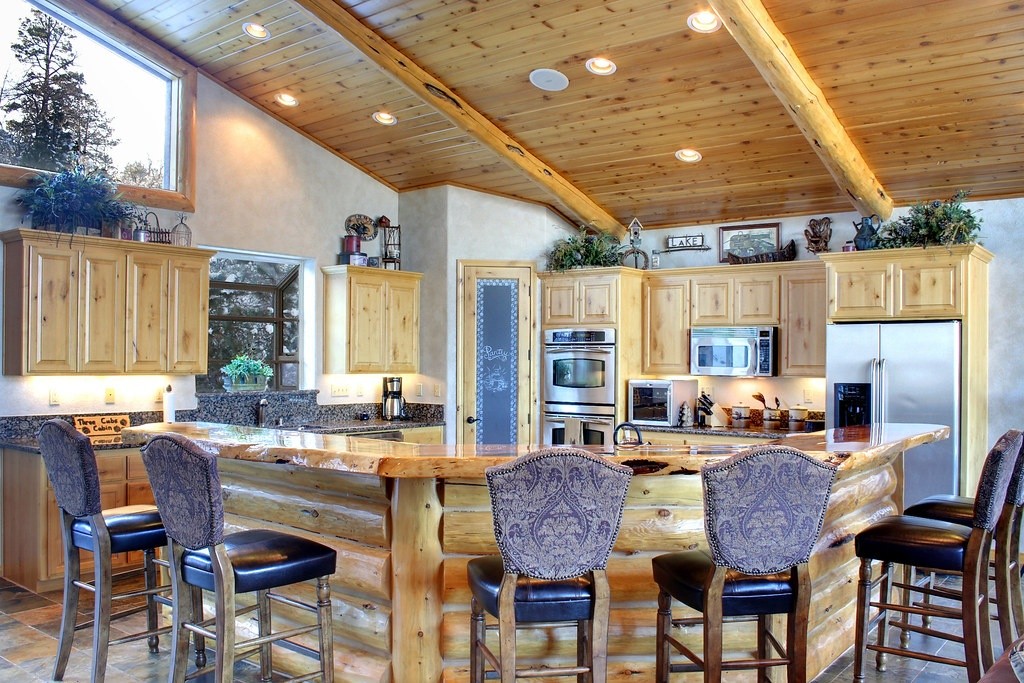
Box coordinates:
[613,422,652,445]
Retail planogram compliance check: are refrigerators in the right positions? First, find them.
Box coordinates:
[824,321,962,512]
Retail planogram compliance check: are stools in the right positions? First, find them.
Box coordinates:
[39,420,837,683]
[853,429,1024,683]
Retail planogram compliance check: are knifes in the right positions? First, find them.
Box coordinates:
[698,393,712,415]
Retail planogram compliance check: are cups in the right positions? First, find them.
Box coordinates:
[789,404,809,431]
[762,409,781,430]
[359,413,369,420]
[732,402,750,428]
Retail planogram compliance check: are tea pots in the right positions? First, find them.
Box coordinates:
[386,394,405,419]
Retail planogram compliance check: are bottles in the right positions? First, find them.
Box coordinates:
[697,391,705,428]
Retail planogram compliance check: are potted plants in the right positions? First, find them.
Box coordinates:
[219,354,274,392]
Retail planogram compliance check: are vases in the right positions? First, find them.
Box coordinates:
[571,266,602,269]
[36,224,101,237]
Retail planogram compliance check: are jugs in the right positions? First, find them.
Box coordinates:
[853,214,881,250]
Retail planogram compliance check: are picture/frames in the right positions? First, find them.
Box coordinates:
[719,223,781,263]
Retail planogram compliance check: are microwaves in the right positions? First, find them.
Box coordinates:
[628,377,698,426]
[690,326,778,378]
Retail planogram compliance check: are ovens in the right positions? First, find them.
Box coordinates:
[543,328,615,446]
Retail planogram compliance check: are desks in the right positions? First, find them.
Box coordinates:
[121,423,950,683]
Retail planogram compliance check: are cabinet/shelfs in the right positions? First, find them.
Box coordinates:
[320,264,425,374]
[817,243,996,322]
[0,444,159,593]
[0,228,217,376]
[400,426,444,445]
[537,266,644,329]
[644,260,826,377]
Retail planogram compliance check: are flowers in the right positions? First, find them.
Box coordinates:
[543,224,632,274]
[13,164,137,256]
[870,188,984,250]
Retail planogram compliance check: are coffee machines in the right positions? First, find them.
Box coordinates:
[381,377,406,422]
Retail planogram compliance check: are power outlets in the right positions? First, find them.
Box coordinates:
[155,387,163,401]
[434,384,440,397]
